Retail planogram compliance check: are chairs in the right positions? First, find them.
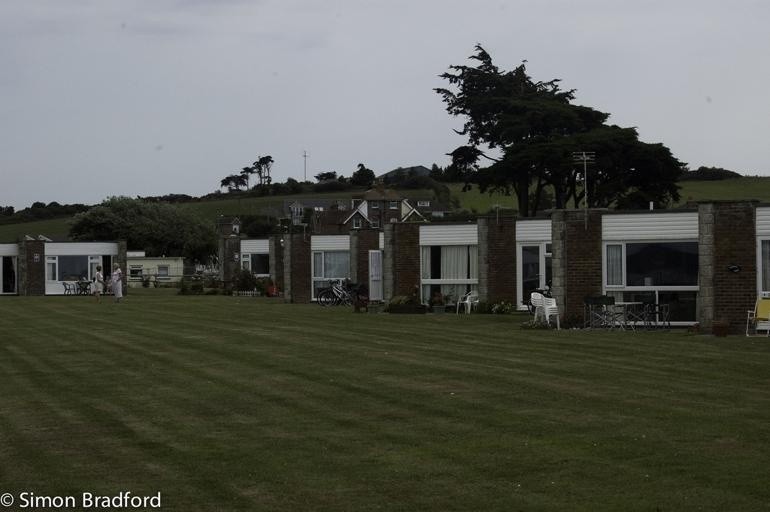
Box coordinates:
[746,296,770,337]
[580,291,671,332]
[63,282,75,296]
[456,290,479,315]
[530,291,560,330]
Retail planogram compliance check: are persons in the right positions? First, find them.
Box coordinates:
[94,266,107,304]
[111,262,123,303]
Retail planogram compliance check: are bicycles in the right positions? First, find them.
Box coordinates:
[527,279,552,315]
[317,278,370,310]
